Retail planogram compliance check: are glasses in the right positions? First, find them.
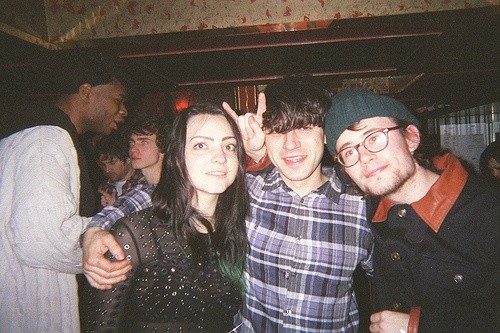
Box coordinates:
[333,123,407,167]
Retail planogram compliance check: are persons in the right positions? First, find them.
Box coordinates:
[83,104,251,333]
[1,56,500,333]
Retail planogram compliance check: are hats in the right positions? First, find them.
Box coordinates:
[324,86,423,156]
